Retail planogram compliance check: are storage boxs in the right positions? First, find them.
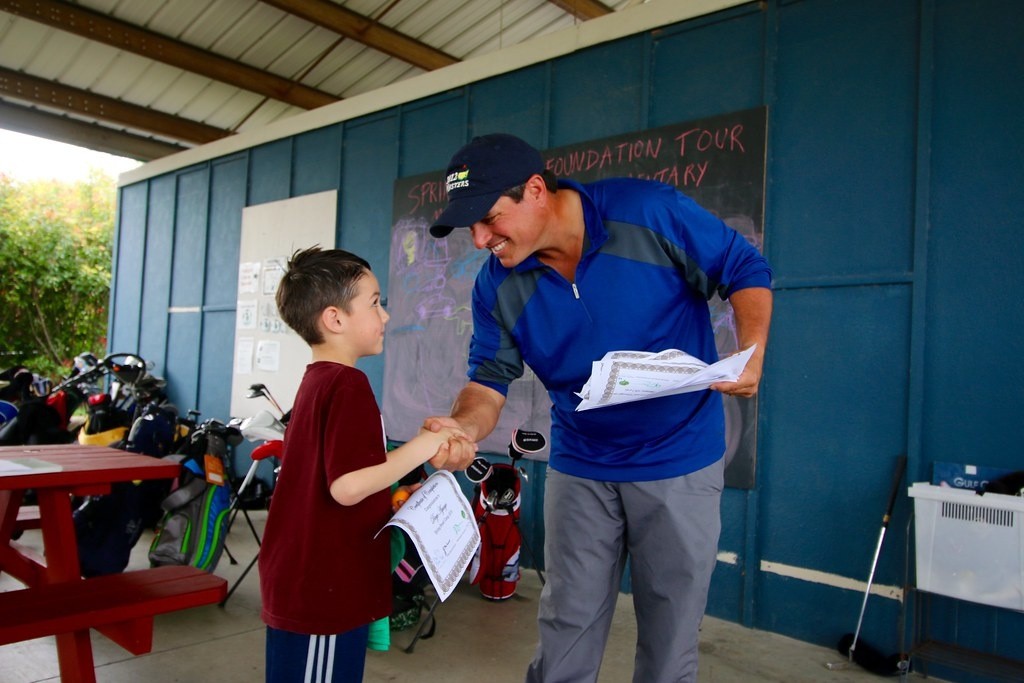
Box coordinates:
[906,481,1024,613]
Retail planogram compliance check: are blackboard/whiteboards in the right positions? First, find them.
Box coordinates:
[379,103,770,490]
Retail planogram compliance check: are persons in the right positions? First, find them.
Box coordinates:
[420,134,774,683]
[256,243,479,683]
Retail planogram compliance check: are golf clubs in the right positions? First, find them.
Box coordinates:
[402,427,547,654]
[826,454,907,669]
[245,380,286,418]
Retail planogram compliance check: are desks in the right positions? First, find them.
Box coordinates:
[0,443,179,683]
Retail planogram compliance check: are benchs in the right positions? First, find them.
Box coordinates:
[0,566,228,645]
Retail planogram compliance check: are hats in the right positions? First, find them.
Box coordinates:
[430,133,544,238]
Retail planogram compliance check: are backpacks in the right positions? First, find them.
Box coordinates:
[147,459,239,574]
[0,385,176,575]
[470,462,522,600]
